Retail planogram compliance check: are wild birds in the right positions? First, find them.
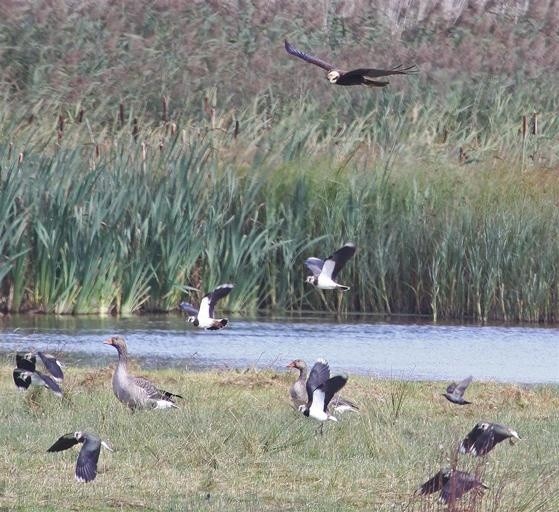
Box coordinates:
[103,336,184,415]
[296,356,349,437]
[286,358,360,413]
[179,284,234,331]
[458,421,522,458]
[302,243,356,293]
[46,431,114,484]
[11,349,67,397]
[441,375,473,405]
[418,467,491,505]
[285,38,420,88]
